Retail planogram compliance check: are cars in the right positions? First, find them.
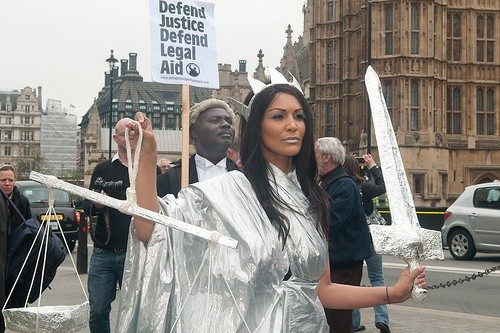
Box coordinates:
[14,180,97,254]
[441,179,500,261]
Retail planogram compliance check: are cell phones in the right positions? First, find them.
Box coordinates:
[355,157,365,163]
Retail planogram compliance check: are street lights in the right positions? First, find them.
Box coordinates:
[105,50,119,159]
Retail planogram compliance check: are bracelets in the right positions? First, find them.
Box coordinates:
[386,287,390,304]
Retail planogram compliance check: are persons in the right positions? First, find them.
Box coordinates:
[158,159,183,174]
[157,99,240,201]
[487,180,500,203]
[315,137,372,333]
[84,117,163,333]
[116,80,426,333]
[0,165,32,333]
[343,152,391,333]
[226,138,243,170]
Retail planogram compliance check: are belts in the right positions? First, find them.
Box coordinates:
[96,245,125,253]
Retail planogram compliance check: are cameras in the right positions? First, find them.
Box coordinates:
[92,176,124,194]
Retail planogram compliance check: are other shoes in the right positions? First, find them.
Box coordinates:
[375,322,391,333]
[353,325,365,332]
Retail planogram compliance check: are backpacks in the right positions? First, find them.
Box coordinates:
[0,188,67,308]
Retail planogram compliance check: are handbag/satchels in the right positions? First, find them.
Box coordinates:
[359,183,386,233]
